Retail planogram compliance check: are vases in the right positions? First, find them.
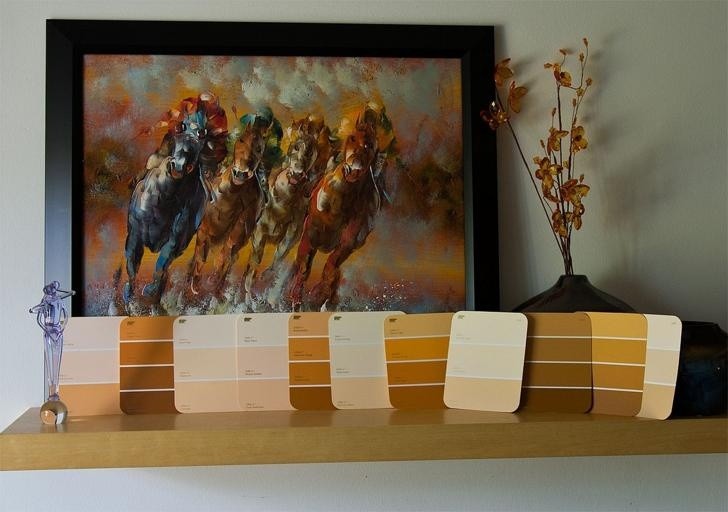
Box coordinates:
[512,275,633,314]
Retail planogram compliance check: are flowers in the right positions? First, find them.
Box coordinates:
[482,38,594,273]
